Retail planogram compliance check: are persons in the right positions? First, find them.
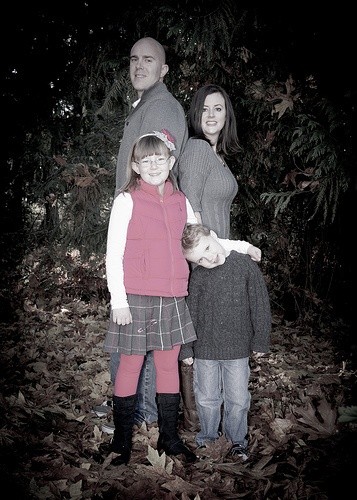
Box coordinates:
[179,83,238,365]
[105,131,261,466]
[180,223,270,463]
[104,37,188,435]
